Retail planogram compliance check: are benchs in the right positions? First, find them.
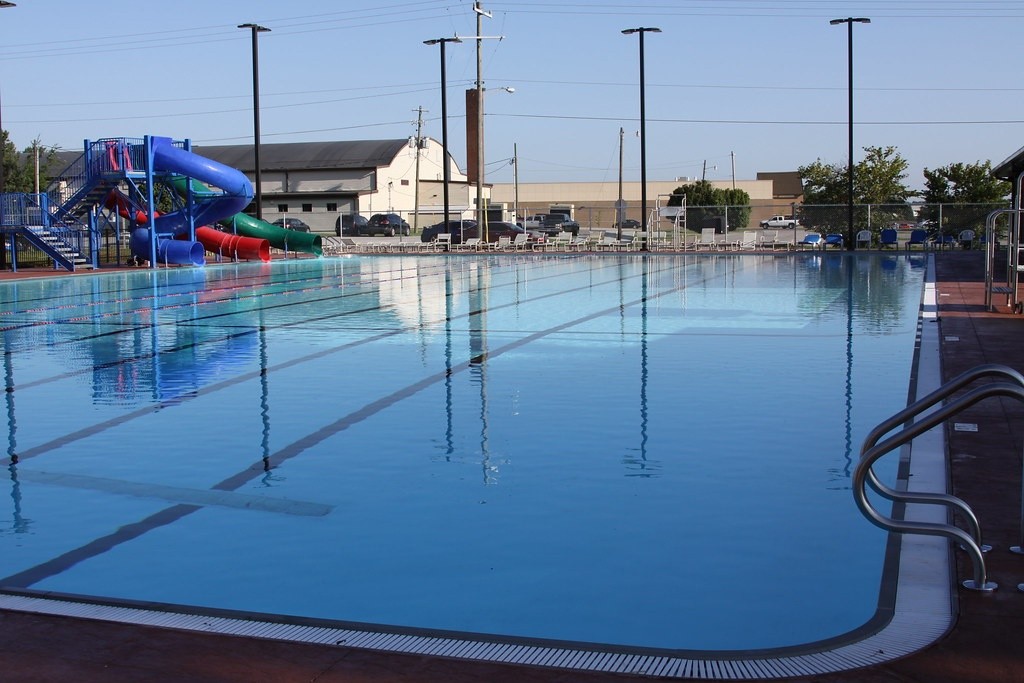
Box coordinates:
[633,232,667,250]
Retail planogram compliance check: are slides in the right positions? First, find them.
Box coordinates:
[93,136,323,268]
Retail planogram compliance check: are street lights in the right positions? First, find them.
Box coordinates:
[236,21,273,222]
[422,32,463,253]
[829,17,873,251]
[621,25,662,252]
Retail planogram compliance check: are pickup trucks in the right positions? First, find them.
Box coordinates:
[759,214,800,230]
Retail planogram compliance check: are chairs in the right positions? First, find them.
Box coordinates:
[532,237,559,251]
[508,234,529,251]
[613,230,635,251]
[978,233,1000,251]
[494,236,511,251]
[932,235,953,249]
[739,230,757,250]
[342,239,434,251]
[524,232,544,251]
[760,230,778,251]
[958,230,975,250]
[717,240,741,250]
[457,238,480,252]
[774,231,794,251]
[595,231,616,252]
[856,230,871,249]
[479,241,495,252]
[823,234,844,251]
[555,232,572,250]
[878,229,898,250]
[905,230,928,250]
[799,234,821,251]
[434,233,451,252]
[695,228,714,251]
[564,236,590,251]
[584,231,604,252]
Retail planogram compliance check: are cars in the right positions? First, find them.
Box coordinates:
[367,214,411,237]
[272,218,311,233]
[421,219,477,244]
[457,221,549,249]
[538,213,580,238]
[514,215,544,229]
[612,219,641,229]
[335,213,372,237]
